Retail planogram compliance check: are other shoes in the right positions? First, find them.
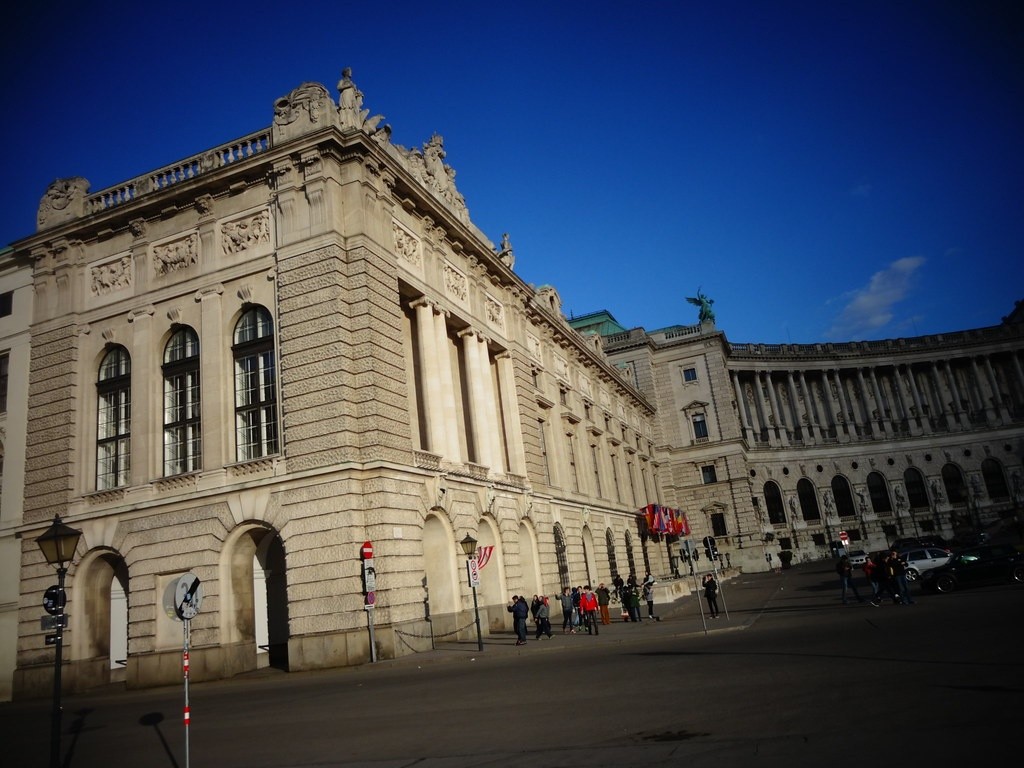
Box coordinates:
[709,614,715,620]
[870,600,880,607]
[892,597,918,606]
[856,596,865,603]
[715,614,721,618]
[513,614,656,646]
[842,599,849,604]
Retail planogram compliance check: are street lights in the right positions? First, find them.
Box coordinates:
[35,513,83,768]
[908,507,922,538]
[460,532,484,651]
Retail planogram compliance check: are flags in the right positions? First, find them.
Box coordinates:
[640,504,691,540]
[477,546,495,569]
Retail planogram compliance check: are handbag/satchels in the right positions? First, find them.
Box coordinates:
[620,607,630,616]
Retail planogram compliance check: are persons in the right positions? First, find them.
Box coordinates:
[555,583,612,636]
[506,595,529,646]
[392,222,420,266]
[154,233,199,274]
[92,257,133,296]
[702,574,720,620]
[614,571,654,623]
[222,210,270,255]
[863,556,883,607]
[885,551,917,605]
[445,266,466,300]
[500,233,512,255]
[837,555,861,605]
[337,64,361,130]
[697,286,714,321]
[530,595,555,641]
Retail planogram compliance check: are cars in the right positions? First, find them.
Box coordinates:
[919,541,1024,593]
[898,548,981,593]
[846,549,869,567]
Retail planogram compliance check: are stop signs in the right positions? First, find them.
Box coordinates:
[362,541,372,559]
[839,531,849,541]
